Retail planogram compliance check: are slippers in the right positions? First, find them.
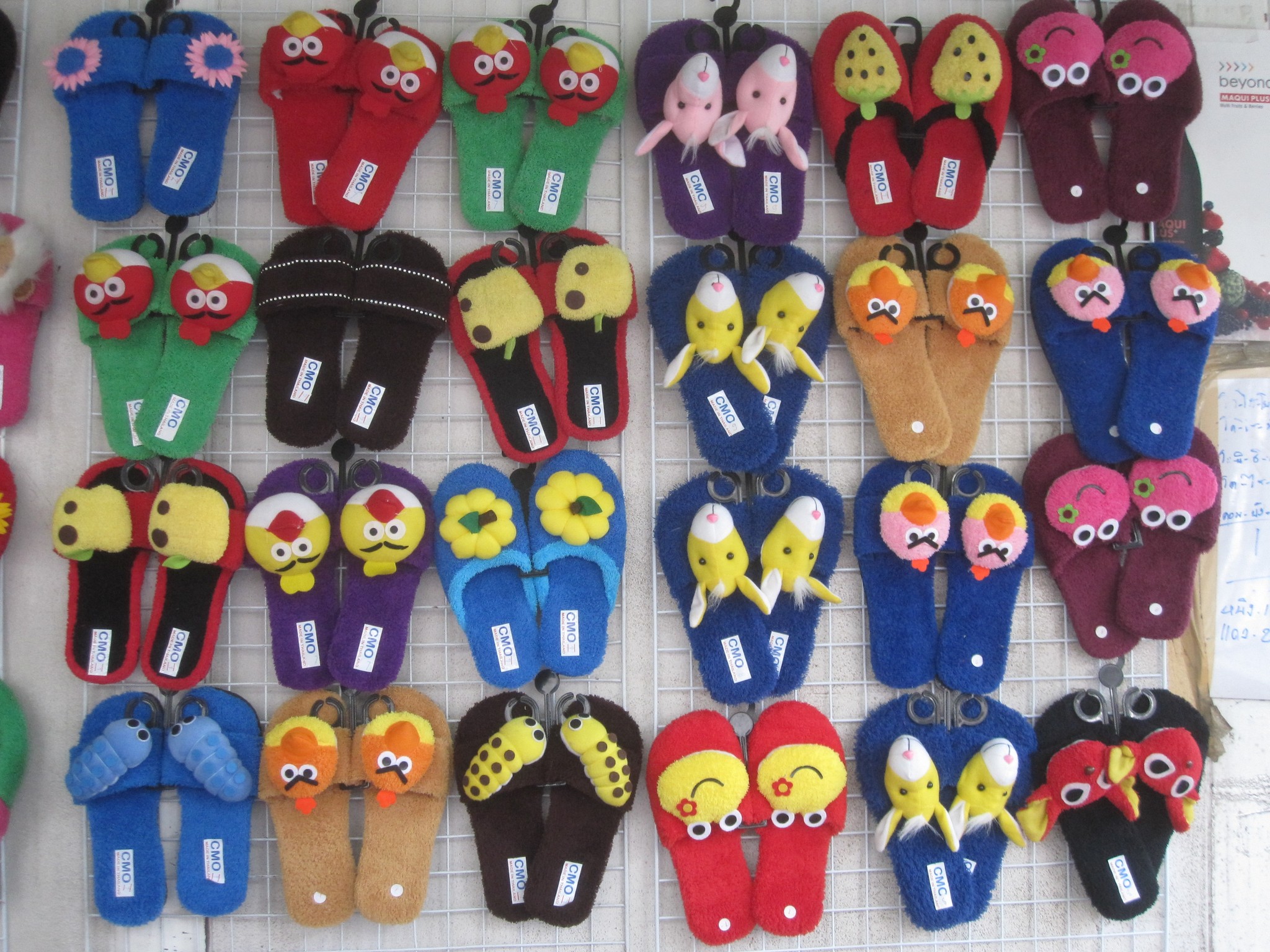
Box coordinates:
[0,3,1230,945]
